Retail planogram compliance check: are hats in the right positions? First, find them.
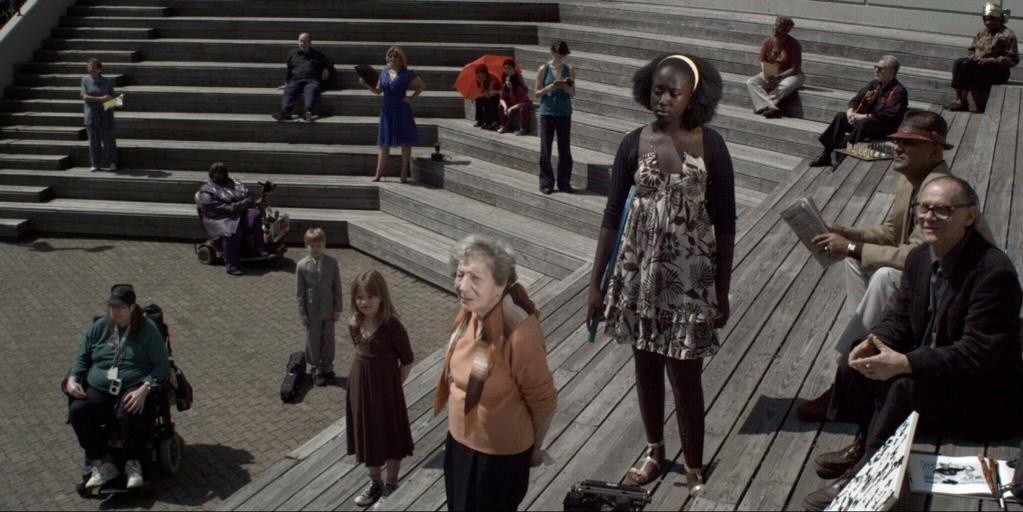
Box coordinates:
[888,110,954,149]
[108,285,135,306]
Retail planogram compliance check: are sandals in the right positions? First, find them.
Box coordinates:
[627,440,666,486]
[683,462,705,499]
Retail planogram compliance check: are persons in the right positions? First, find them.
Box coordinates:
[65,283,169,488]
[294,227,342,386]
[196,162,262,276]
[470,63,501,129]
[78,58,119,173]
[585,52,736,498]
[799,176,1023,512]
[493,70,531,135]
[534,40,577,195]
[342,268,416,507]
[356,44,428,185]
[434,233,556,511]
[943,2,1021,111]
[795,112,994,422]
[808,54,906,168]
[745,14,804,117]
[501,57,528,94]
[271,33,339,123]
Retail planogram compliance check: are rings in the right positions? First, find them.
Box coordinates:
[864,362,871,369]
[825,245,827,254]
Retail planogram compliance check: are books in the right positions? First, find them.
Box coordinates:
[102,92,123,111]
[761,60,780,83]
[910,452,1018,503]
[552,78,574,92]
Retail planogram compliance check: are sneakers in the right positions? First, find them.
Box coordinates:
[85,462,120,488]
[126,460,146,488]
[809,156,831,166]
[950,97,970,110]
[763,108,781,118]
[497,128,506,133]
[311,372,336,386]
[355,480,399,511]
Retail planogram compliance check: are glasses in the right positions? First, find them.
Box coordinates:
[910,202,974,220]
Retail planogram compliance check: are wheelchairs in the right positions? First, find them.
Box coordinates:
[65,305,193,498]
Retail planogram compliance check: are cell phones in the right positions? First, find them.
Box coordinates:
[108,378,122,396]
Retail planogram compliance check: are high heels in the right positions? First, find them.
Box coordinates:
[371,168,386,182]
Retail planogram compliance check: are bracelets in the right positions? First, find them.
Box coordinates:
[144,382,153,390]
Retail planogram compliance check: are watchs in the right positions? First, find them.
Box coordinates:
[845,239,857,257]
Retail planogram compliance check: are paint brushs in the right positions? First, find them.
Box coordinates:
[978,453,1005,509]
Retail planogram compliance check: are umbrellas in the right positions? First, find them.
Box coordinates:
[453,54,523,100]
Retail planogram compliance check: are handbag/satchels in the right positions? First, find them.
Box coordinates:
[167,363,192,412]
[562,480,652,511]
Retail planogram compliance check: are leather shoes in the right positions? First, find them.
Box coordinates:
[797,391,832,421]
[803,477,851,511]
[814,445,864,477]
